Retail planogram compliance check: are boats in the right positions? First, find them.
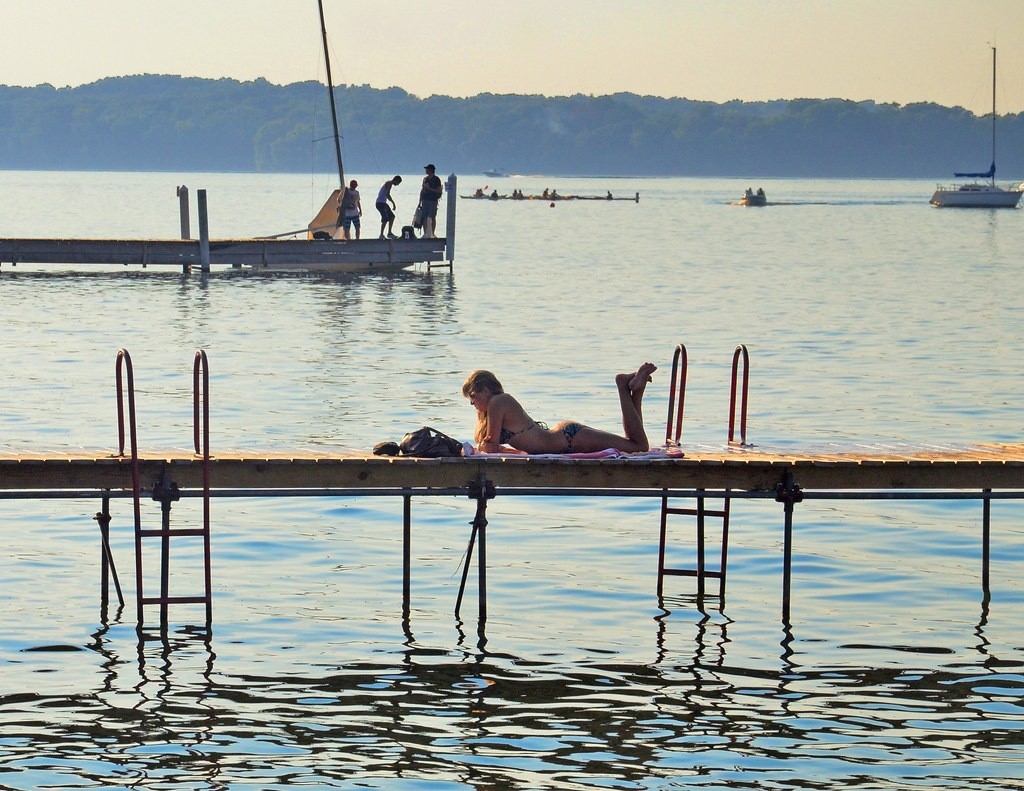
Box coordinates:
[459,194,641,201]
[742,193,766,206]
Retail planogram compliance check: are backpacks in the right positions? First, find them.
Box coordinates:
[412,205,424,229]
[397,226,418,240]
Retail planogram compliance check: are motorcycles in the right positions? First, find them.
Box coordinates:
[482,169,504,177]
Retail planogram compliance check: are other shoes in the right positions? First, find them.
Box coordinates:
[379,235,387,240]
[387,233,398,239]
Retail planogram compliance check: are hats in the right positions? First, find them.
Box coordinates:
[351,180,358,188]
[424,163,436,171]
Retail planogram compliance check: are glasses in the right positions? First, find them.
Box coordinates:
[469,389,482,401]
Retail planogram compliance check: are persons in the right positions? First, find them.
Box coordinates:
[513,190,523,198]
[748,188,753,195]
[607,191,612,199]
[758,188,763,195]
[476,189,483,197]
[463,363,657,455]
[543,188,549,198]
[491,190,498,197]
[337,180,362,240]
[376,175,402,239]
[418,164,442,238]
[551,190,557,198]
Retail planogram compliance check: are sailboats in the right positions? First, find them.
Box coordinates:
[930,46,1024,208]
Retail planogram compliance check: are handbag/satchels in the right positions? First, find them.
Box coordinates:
[394,427,463,459]
